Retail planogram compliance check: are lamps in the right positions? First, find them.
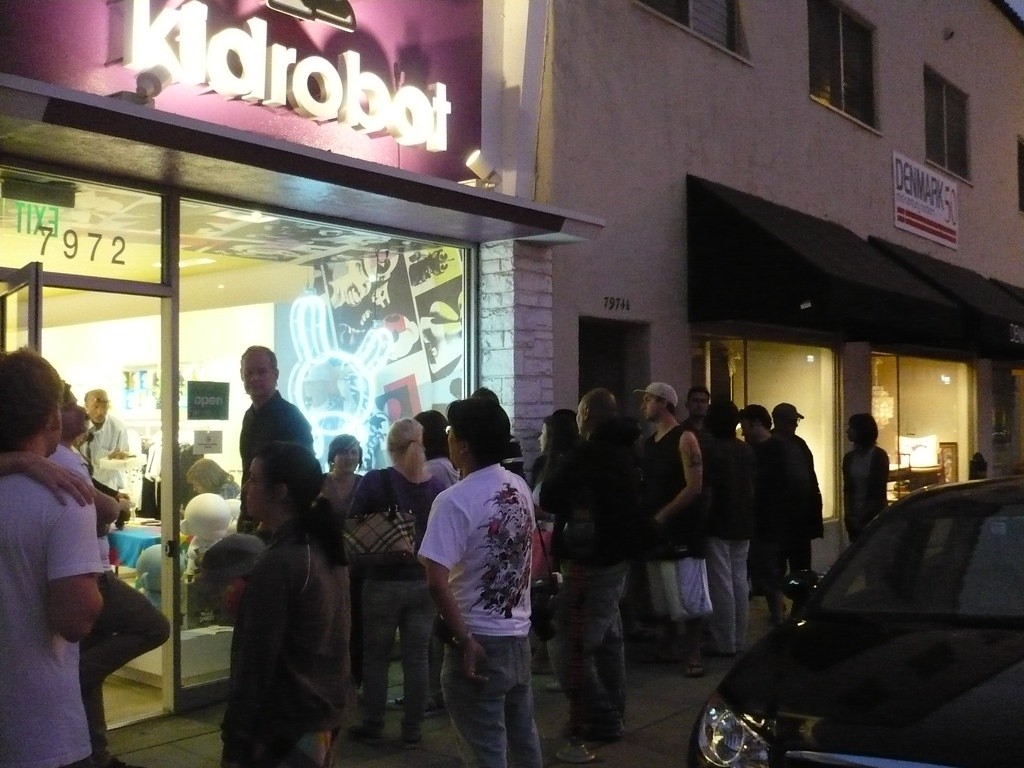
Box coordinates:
[872,358,895,431]
[459,149,502,192]
[265,0,356,33]
[106,63,173,108]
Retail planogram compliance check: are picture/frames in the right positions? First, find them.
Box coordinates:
[939,442,958,483]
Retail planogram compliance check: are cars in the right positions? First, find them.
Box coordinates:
[683,473,1024,768]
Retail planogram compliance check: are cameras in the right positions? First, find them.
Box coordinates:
[530,575,559,641]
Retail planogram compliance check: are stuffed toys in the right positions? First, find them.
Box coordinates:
[133,541,182,594]
[180,492,243,582]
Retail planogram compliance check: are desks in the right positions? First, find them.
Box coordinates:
[110,521,162,566]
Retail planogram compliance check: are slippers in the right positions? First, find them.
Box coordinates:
[685,656,705,676]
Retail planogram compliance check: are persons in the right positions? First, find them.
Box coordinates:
[844,412,888,537]
[237,346,315,533]
[0,348,172,768]
[970,451,986,481]
[221,383,825,768]
[173,438,240,507]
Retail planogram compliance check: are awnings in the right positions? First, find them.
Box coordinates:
[867,231,1023,362]
[687,174,964,338]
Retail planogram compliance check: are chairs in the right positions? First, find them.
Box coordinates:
[896,434,945,490]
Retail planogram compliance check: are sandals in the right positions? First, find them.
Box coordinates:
[424,696,444,712]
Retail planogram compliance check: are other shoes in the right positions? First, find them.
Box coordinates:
[625,627,655,642]
[530,654,553,673]
[703,648,736,658]
[546,679,563,693]
[347,723,382,744]
[403,736,422,749]
[396,695,406,704]
[565,716,626,740]
[107,758,147,768]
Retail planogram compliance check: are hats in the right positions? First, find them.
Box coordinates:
[634,382,678,407]
[772,403,804,420]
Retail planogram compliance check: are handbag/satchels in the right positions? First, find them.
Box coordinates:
[343,472,416,566]
[529,570,559,642]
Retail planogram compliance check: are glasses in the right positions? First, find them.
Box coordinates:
[445,425,454,435]
